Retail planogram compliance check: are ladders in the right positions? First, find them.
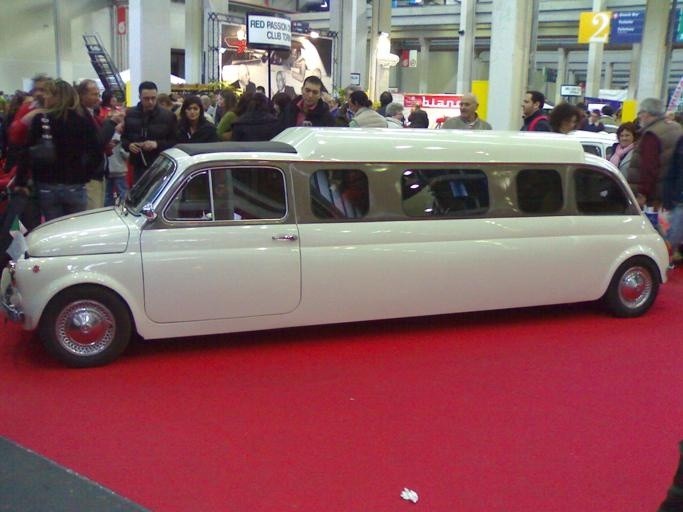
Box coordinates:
[82,32,127,102]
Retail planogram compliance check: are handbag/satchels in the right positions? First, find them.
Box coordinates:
[29,142,55,170]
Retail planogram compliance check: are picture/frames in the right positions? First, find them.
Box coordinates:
[351,73,360,86]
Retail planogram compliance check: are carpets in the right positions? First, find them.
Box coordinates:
[0,269,683,512]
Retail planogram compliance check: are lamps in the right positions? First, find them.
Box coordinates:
[310,30,320,39]
[377,32,400,68]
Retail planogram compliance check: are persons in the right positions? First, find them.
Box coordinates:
[442,94,493,130]
[1,75,428,278]
[518,90,683,271]
